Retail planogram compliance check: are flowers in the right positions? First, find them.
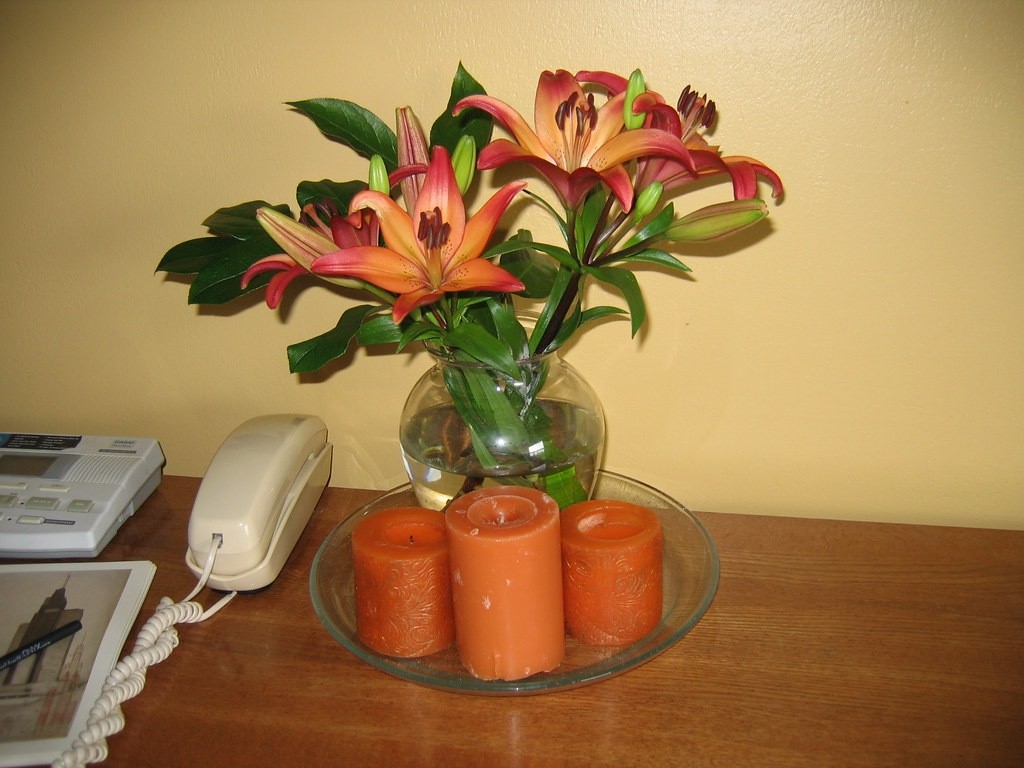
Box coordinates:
[154,63,783,381]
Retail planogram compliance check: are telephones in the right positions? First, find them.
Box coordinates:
[183,413,335,593]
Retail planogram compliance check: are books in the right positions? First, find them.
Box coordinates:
[0,560,157,767]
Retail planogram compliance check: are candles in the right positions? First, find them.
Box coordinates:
[559,499,665,649]
[351,506,454,659]
[446,485,566,684]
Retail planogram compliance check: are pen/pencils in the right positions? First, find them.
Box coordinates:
[0,620,83,673]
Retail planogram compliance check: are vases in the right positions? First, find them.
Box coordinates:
[399,316,608,510]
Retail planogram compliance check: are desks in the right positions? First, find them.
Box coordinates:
[0,475,1024,768]
